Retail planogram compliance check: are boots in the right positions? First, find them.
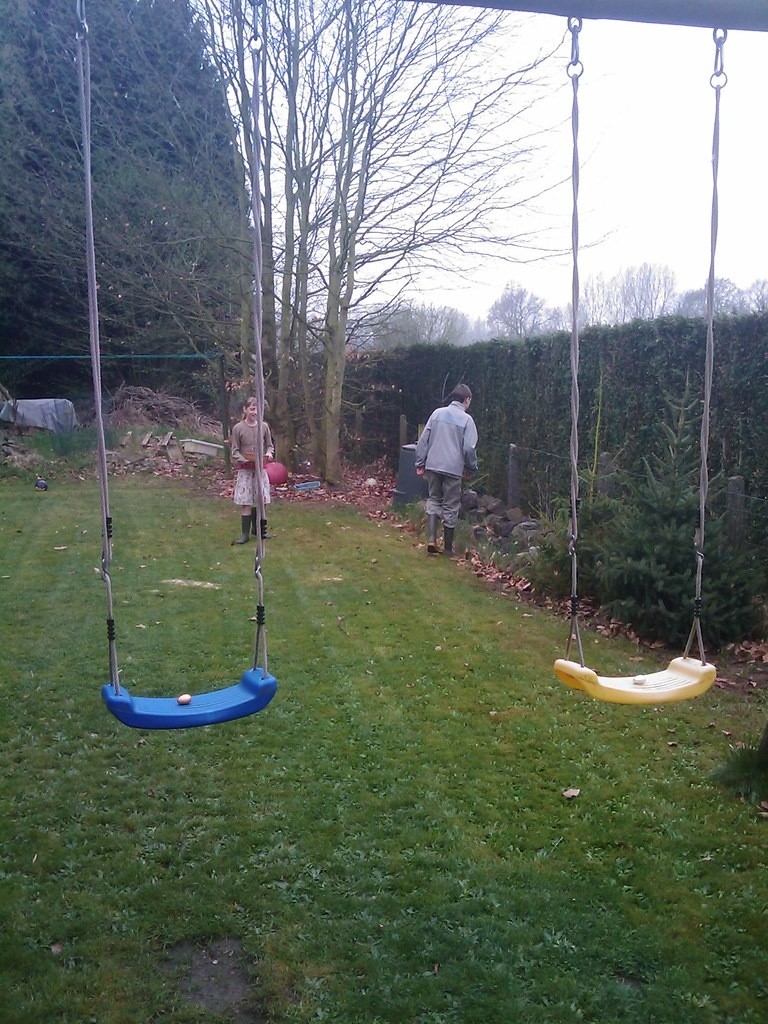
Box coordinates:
[427,514,438,552]
[444,524,455,556]
[251,507,257,536]
[236,515,252,543]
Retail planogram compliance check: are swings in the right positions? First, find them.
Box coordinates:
[554,12,729,705]
[74,0,279,732]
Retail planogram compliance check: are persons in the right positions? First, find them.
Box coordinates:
[231,397,274,544]
[415,384,478,557]
